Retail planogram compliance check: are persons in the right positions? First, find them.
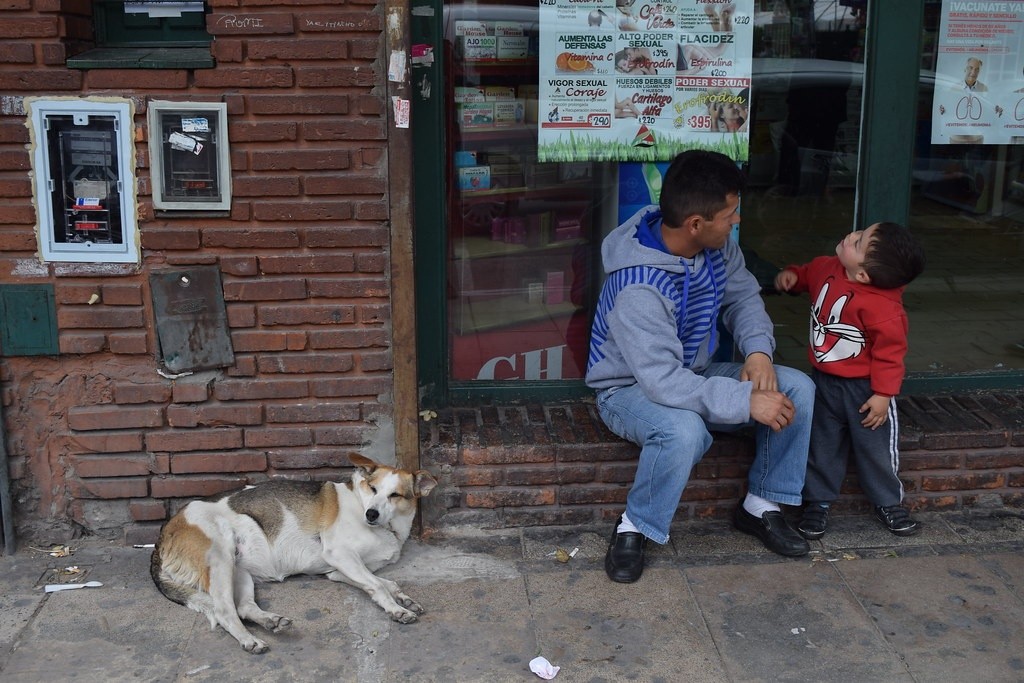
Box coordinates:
[940,57,1024,144]
[773,222,917,538]
[584,150,816,584]
[615,0,749,132]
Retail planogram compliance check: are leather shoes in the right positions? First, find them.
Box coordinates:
[605,515,648,584]
[734,499,817,556]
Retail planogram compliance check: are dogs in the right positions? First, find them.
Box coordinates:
[149,452,439,655]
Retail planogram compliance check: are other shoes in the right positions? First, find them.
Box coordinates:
[792,501,829,539]
[880,509,920,535]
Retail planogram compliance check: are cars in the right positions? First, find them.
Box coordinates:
[738,56,1023,227]
